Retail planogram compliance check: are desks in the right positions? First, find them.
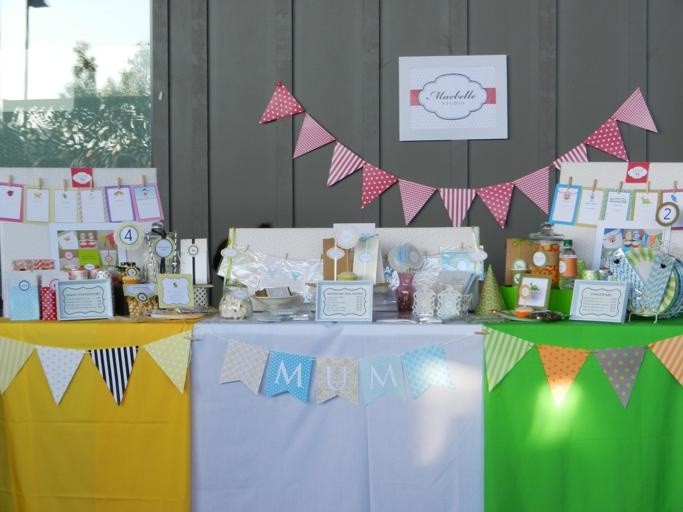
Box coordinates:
[0,315,682,512]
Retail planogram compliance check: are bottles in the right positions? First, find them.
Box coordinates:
[527,221,564,290]
[557,239,577,290]
[117,261,141,283]
[394,271,416,318]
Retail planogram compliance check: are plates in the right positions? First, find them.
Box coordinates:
[149,314,204,319]
[486,308,538,321]
[617,251,683,317]
[250,295,296,310]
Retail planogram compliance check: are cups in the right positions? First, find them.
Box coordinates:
[598,267,611,280]
[69,269,87,280]
[510,269,527,289]
[581,270,599,281]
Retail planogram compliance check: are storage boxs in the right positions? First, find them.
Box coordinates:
[501,237,573,289]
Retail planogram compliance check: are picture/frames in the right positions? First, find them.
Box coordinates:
[396,54,510,143]
[54,278,114,320]
[569,278,632,324]
[315,279,374,323]
[591,219,673,277]
[513,273,554,311]
